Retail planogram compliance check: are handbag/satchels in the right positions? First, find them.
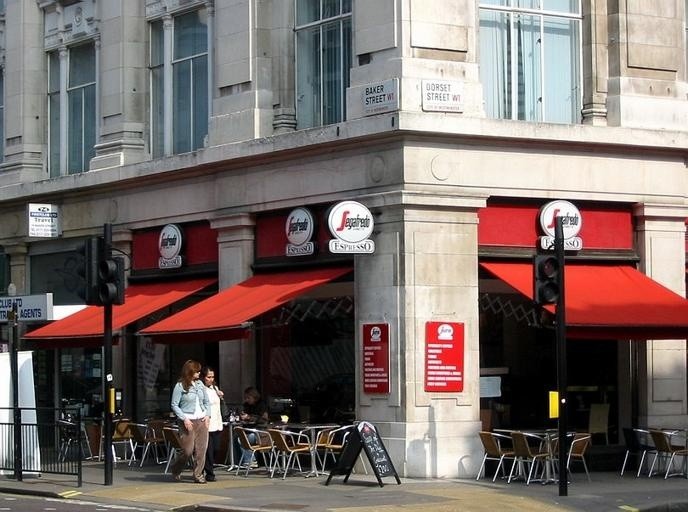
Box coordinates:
[220,398,230,418]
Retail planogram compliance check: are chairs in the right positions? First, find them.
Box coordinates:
[620,427,688,480]
[58,419,208,480]
[476,429,593,486]
[233,419,368,481]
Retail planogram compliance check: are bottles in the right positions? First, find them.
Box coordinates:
[228,407,240,423]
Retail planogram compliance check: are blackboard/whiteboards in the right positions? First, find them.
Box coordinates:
[357,420,396,477]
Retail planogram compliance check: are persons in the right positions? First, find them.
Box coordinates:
[167,359,211,485]
[239,387,270,470]
[200,364,225,483]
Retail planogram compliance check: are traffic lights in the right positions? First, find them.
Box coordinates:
[75,235,100,306]
[97,257,128,305]
[531,251,560,308]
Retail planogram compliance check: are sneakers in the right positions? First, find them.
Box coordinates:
[244,461,258,468]
[171,465,181,482]
[195,477,205,483]
